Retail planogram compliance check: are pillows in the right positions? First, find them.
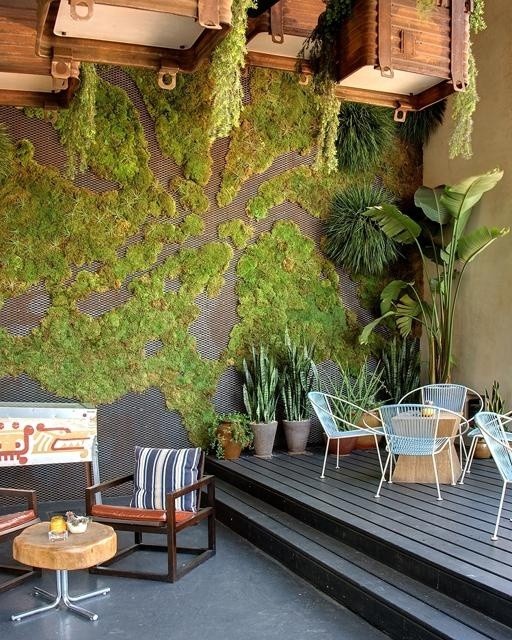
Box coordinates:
[131,446,202,514]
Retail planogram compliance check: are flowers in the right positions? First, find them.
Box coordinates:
[65,511,89,527]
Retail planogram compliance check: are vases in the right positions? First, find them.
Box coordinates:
[66,522,87,533]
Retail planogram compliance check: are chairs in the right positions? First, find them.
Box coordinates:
[85,450,216,582]
[305,384,512,541]
[0,487,41,594]
